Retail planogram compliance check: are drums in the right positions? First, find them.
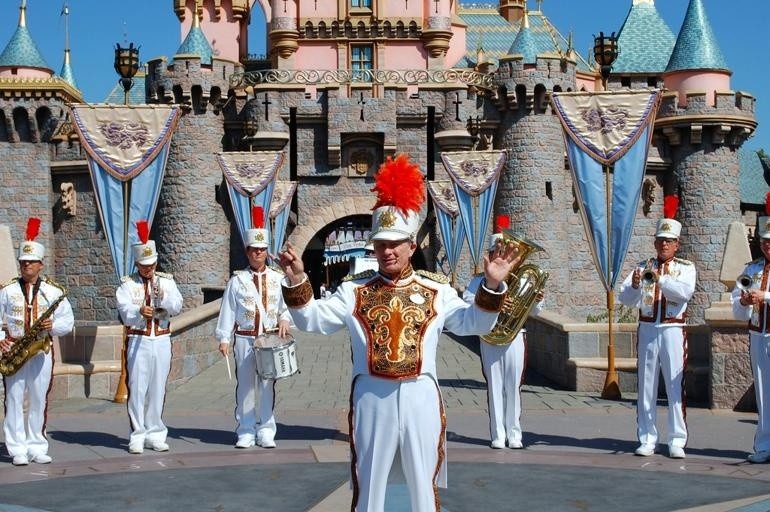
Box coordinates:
[252,332,300,381]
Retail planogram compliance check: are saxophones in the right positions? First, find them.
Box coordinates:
[0,274,72,377]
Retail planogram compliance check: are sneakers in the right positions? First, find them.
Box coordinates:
[490,440,506,449]
[635,443,660,456]
[747,450,769,462]
[145,439,169,451]
[12,455,29,465]
[508,440,523,449]
[257,438,276,447]
[236,438,256,448]
[32,454,53,464]
[128,444,144,453]
[668,447,686,458]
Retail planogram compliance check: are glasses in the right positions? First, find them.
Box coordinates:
[655,237,676,244]
[759,237,770,243]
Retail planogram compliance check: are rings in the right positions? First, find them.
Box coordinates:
[289,258,295,265]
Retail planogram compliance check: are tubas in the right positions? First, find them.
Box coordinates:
[478,224,549,346]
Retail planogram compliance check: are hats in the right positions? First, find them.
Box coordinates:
[656,218,682,239]
[245,228,269,249]
[487,233,505,252]
[132,240,158,265]
[17,241,45,263]
[369,205,420,245]
[758,216,770,238]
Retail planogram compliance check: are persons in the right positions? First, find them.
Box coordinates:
[618,217,697,458]
[115,239,183,454]
[320,283,326,299]
[0,240,74,466]
[214,228,292,448]
[268,205,521,512]
[462,233,544,449]
[731,216,770,464]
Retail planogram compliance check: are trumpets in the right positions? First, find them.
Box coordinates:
[148,274,168,318]
[736,274,760,292]
[641,257,657,285]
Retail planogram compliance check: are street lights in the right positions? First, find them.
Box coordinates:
[590,30,621,400]
[110,42,142,406]
